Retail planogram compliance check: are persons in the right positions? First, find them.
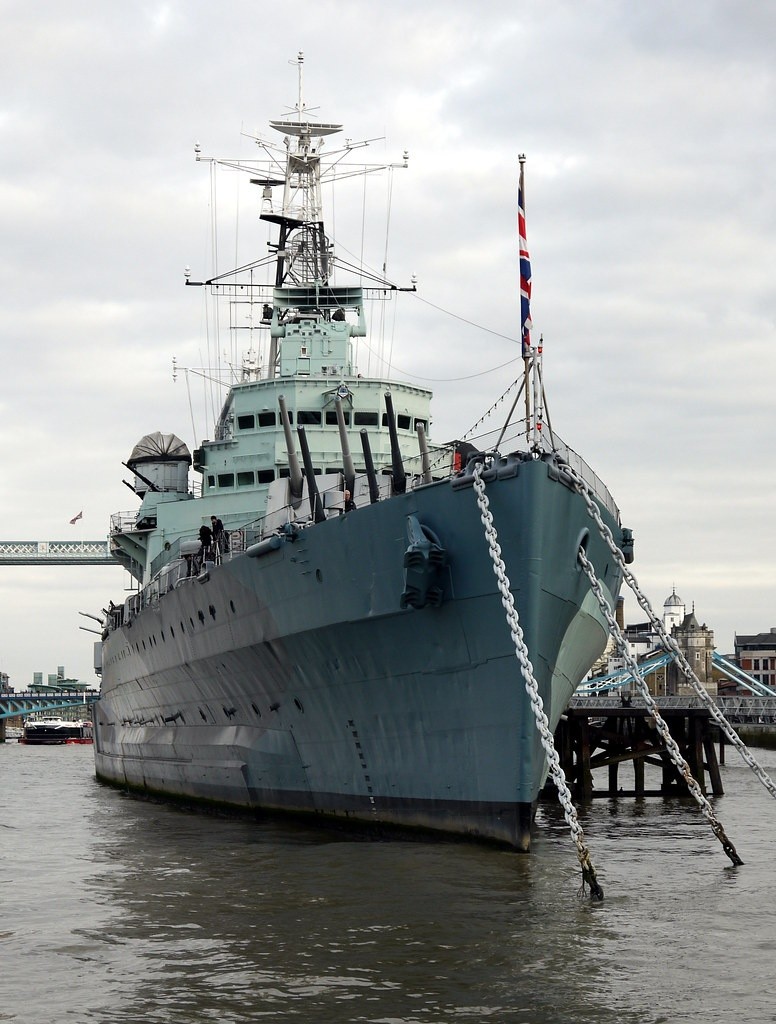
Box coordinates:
[344,490,357,516]
[184,515,225,578]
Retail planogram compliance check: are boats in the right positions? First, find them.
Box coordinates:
[76,47,637,856]
[16,714,94,746]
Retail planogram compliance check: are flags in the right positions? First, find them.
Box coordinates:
[517,164,533,360]
[70,511,82,524]
